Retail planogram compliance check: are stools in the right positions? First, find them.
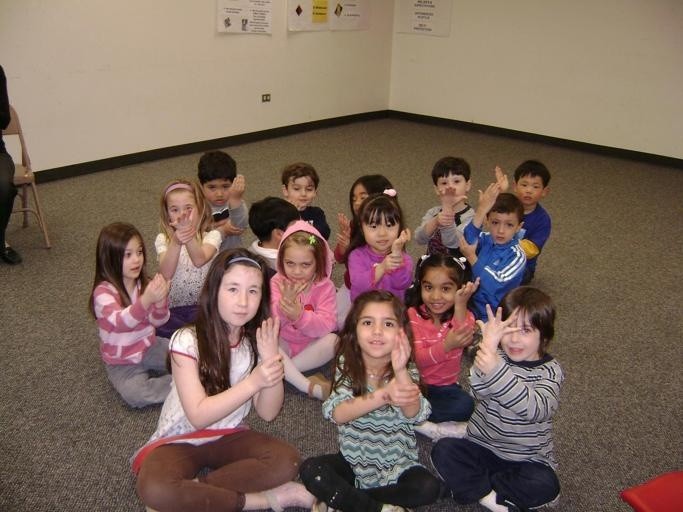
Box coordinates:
[620,471,683,512]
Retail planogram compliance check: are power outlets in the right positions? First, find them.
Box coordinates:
[262,94,271,102]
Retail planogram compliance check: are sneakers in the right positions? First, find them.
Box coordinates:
[309,371,334,401]
[432,420,470,443]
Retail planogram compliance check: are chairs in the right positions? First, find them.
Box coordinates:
[2,105,52,250]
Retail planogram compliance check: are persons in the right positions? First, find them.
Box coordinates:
[88,150,565,511]
[0,65,22,265]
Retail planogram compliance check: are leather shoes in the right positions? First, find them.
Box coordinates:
[3,245,21,264]
